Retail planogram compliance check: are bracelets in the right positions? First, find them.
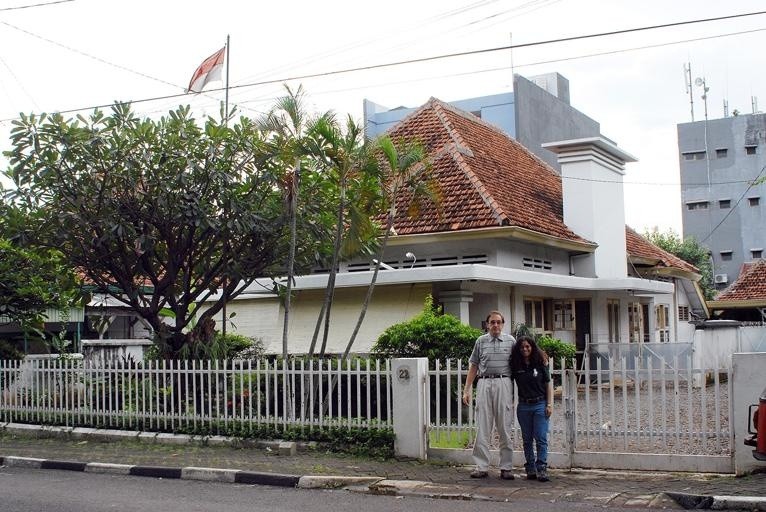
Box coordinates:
[547,404,554,408]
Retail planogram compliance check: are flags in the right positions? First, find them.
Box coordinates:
[184,46,228,98]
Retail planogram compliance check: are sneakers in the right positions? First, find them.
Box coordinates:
[527,470,550,482]
[501,470,515,479]
[470,470,489,478]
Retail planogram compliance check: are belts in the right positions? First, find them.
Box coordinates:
[519,394,545,404]
[477,374,509,378]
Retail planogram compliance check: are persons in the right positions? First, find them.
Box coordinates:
[460,309,550,480]
[509,335,556,481]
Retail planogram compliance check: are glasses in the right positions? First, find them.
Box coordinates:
[488,319,503,324]
